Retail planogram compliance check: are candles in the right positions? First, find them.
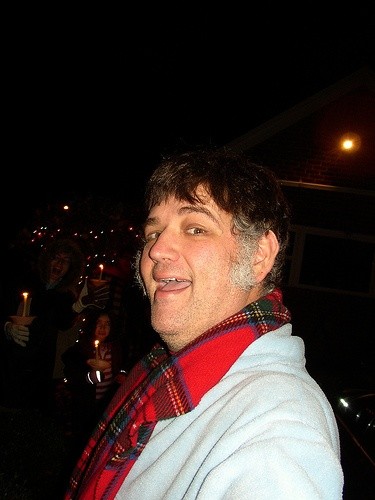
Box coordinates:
[22,292,28,316]
[95,346,100,369]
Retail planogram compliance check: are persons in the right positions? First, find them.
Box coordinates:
[2,239,125,423]
[58,145,346,500]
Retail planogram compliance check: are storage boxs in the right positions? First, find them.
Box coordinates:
[277,179,375,301]
